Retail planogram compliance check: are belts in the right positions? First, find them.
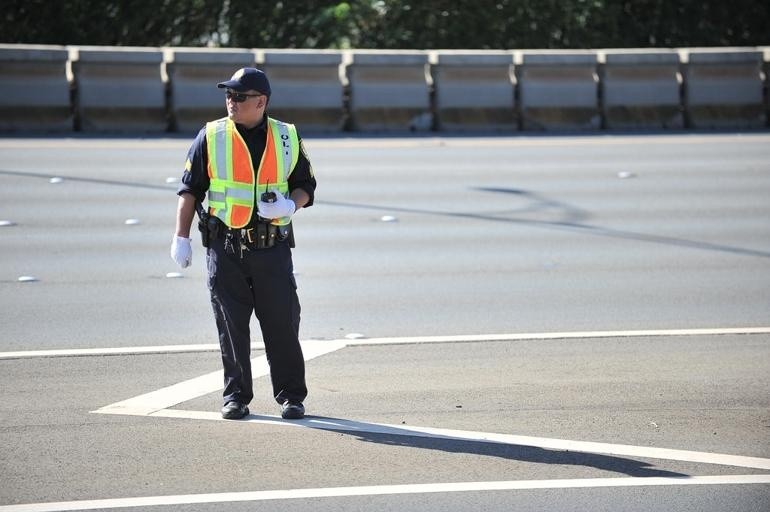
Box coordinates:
[225,227,256,244]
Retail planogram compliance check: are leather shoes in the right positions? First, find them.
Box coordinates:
[281,399,306,419]
[221,400,250,420]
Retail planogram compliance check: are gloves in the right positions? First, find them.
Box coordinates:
[255,187,297,222]
[170,231,194,269]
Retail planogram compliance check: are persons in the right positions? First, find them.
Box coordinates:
[169,68,318,419]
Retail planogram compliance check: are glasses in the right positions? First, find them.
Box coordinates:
[224,90,261,104]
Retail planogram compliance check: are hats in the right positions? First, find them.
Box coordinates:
[217,64,272,97]
